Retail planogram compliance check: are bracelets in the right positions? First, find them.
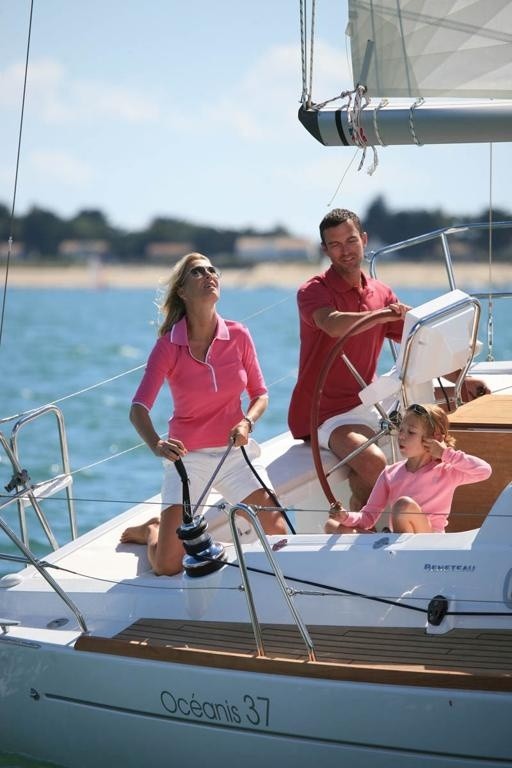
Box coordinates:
[244,416,255,433]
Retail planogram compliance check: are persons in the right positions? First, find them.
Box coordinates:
[287,208,492,446]
[119,252,286,577]
[324,403,492,534]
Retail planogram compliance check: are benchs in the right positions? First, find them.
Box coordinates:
[86,359,511,534]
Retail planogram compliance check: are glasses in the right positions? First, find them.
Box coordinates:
[181,266,216,286]
[406,403,435,430]
